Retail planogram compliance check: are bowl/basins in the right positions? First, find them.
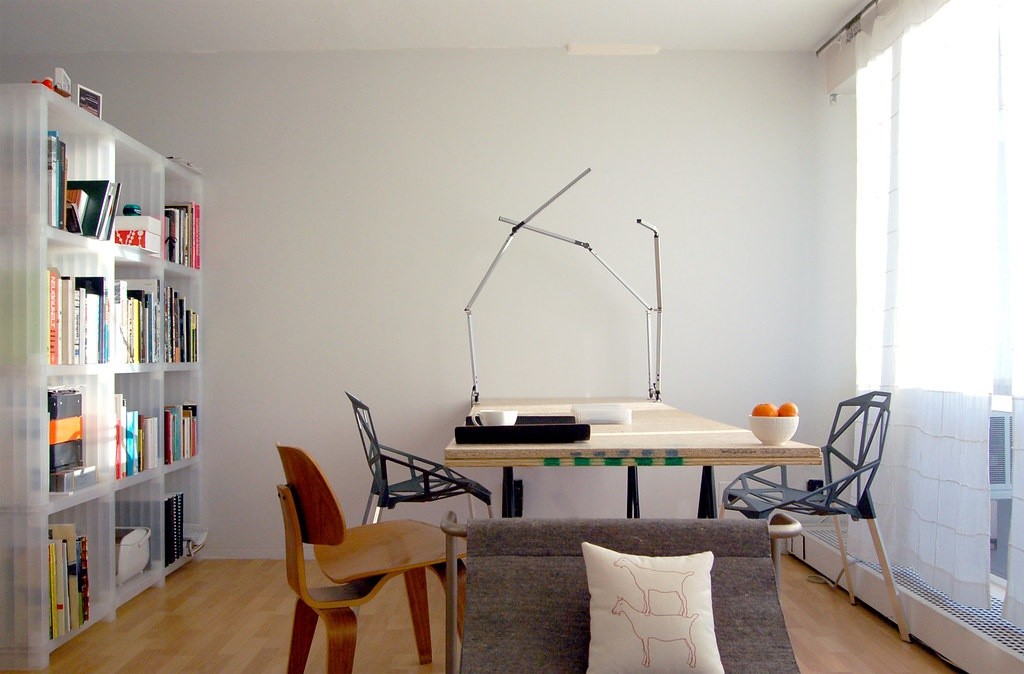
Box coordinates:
[748,414,799,445]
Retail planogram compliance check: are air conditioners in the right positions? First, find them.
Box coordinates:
[983,410,1016,504]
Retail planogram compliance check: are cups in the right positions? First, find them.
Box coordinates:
[472,409,518,426]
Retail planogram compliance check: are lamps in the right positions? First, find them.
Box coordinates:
[499,216,675,402]
[463,168,595,407]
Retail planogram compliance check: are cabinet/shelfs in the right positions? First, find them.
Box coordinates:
[0,84,209,672]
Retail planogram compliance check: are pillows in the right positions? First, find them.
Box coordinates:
[582,543,726,674]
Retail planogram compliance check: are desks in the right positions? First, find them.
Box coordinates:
[444,397,822,519]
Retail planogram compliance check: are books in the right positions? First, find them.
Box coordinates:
[48,129,201,638]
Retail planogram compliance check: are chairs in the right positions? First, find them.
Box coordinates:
[719,391,917,646]
[345,390,496,527]
[442,511,805,674]
[270,441,463,674]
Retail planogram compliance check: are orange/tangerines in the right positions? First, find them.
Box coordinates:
[752,401,798,417]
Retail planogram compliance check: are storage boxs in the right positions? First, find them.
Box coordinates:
[114,216,162,253]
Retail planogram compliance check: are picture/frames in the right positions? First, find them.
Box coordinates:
[78,83,103,120]
[54,68,71,100]
